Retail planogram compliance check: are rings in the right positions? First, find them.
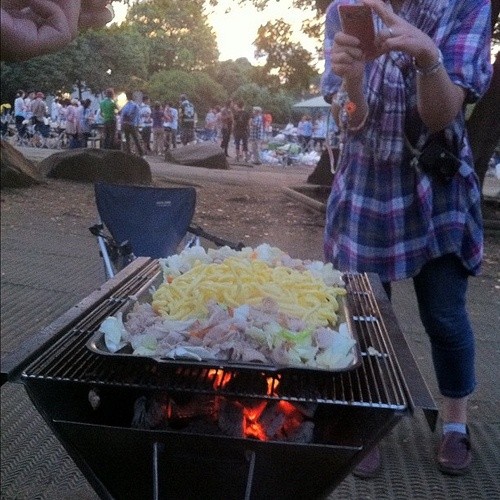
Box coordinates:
[389,28,395,37]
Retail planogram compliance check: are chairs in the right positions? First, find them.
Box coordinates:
[89,183,242,282]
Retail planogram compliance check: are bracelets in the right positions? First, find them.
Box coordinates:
[413,49,443,76]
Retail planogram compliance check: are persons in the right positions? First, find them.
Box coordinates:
[264,110,335,152]
[140,96,153,153]
[320,0,492,478]
[203,107,219,142]
[164,102,178,152]
[219,99,234,157]
[100,88,119,150]
[0,90,107,151]
[179,94,196,146]
[149,101,170,156]
[119,91,147,156]
[232,101,252,163]
[247,107,263,165]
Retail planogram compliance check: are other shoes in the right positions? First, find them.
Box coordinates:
[436,424,472,474]
[353,443,381,479]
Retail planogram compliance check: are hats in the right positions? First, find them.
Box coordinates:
[36,92,44,98]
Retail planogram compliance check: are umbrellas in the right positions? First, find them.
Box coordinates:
[292,95,331,108]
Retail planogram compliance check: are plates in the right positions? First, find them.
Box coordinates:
[88,258,364,372]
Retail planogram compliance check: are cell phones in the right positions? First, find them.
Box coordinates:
[337,4,378,59]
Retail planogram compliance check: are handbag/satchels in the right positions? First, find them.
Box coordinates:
[65,122,76,135]
[29,116,36,125]
